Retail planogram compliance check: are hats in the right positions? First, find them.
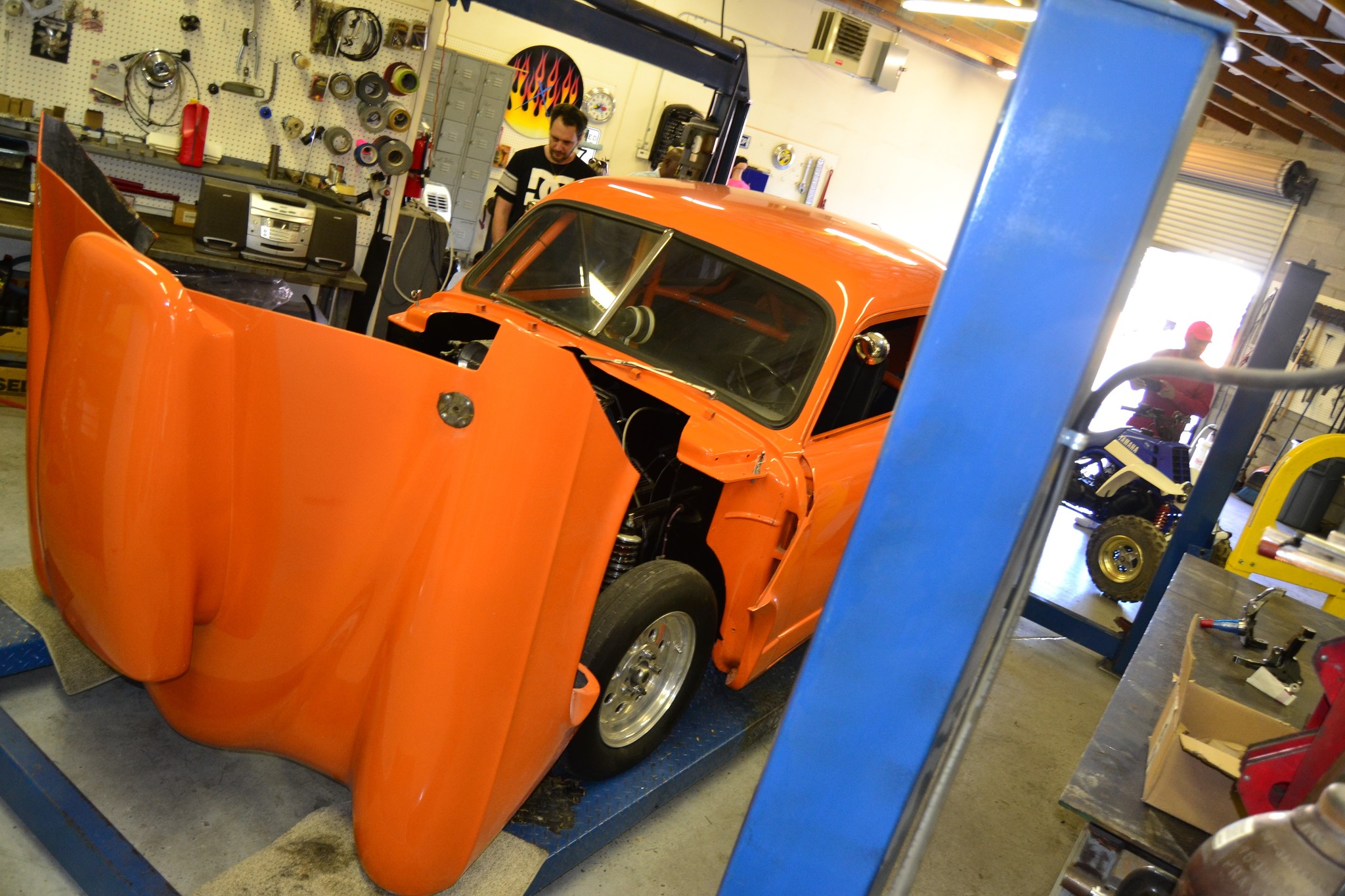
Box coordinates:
[1186,321,1213,343]
[733,156,747,167]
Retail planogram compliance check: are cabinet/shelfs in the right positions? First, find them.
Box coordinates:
[0,125,366,393]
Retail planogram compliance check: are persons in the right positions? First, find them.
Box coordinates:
[1075,321,1216,530]
[726,156,752,189]
[489,104,683,298]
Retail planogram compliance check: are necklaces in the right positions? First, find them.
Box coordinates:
[551,151,572,179]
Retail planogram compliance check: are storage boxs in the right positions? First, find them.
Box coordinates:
[1142,614,1302,837]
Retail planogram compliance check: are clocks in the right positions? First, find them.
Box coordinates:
[584,87,615,123]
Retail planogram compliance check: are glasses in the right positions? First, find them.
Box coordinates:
[588,158,608,176]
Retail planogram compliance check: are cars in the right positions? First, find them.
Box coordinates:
[20,98,949,896]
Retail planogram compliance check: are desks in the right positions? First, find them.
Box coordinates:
[1049,551,1345,896]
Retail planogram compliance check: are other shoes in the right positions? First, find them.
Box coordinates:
[1075,517,1101,528]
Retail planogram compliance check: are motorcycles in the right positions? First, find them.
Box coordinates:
[1063,397,1237,604]
